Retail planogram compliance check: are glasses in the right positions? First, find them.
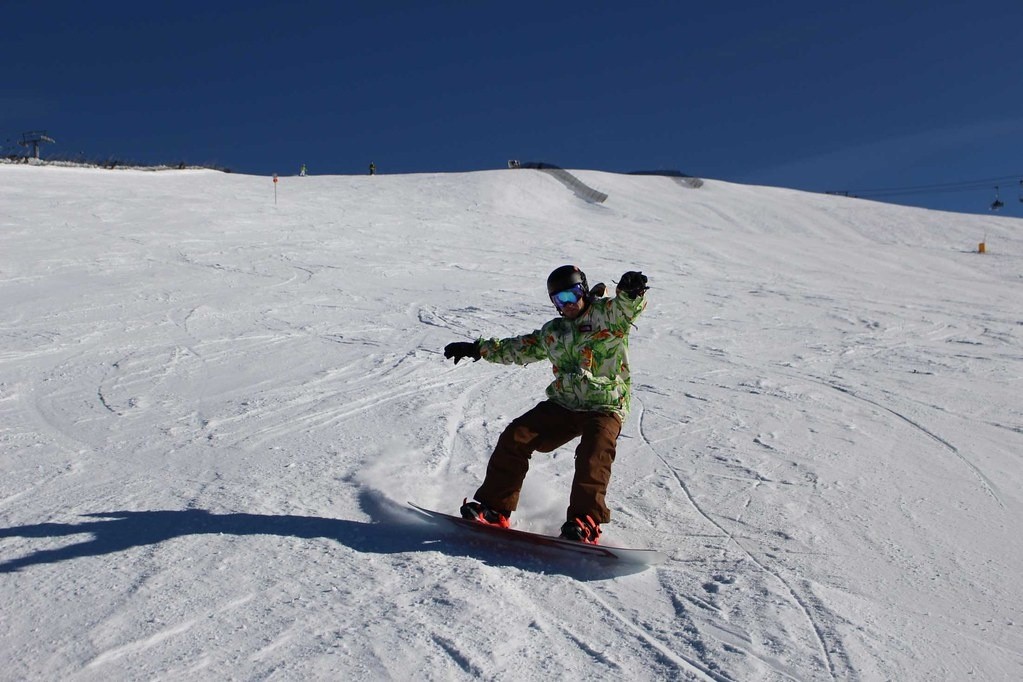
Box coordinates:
[551,284,584,307]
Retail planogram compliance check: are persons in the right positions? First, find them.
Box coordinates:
[444,265,650,545]
[299,164,308,175]
[369,161,376,175]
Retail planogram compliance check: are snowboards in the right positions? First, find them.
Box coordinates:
[408,500,670,566]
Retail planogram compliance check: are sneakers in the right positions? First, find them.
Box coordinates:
[461,498,510,529]
[561,515,602,543]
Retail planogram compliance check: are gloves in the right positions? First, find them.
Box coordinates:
[617,272,648,299]
[444,341,481,365]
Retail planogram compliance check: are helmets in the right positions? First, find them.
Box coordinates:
[547,265,589,311]
